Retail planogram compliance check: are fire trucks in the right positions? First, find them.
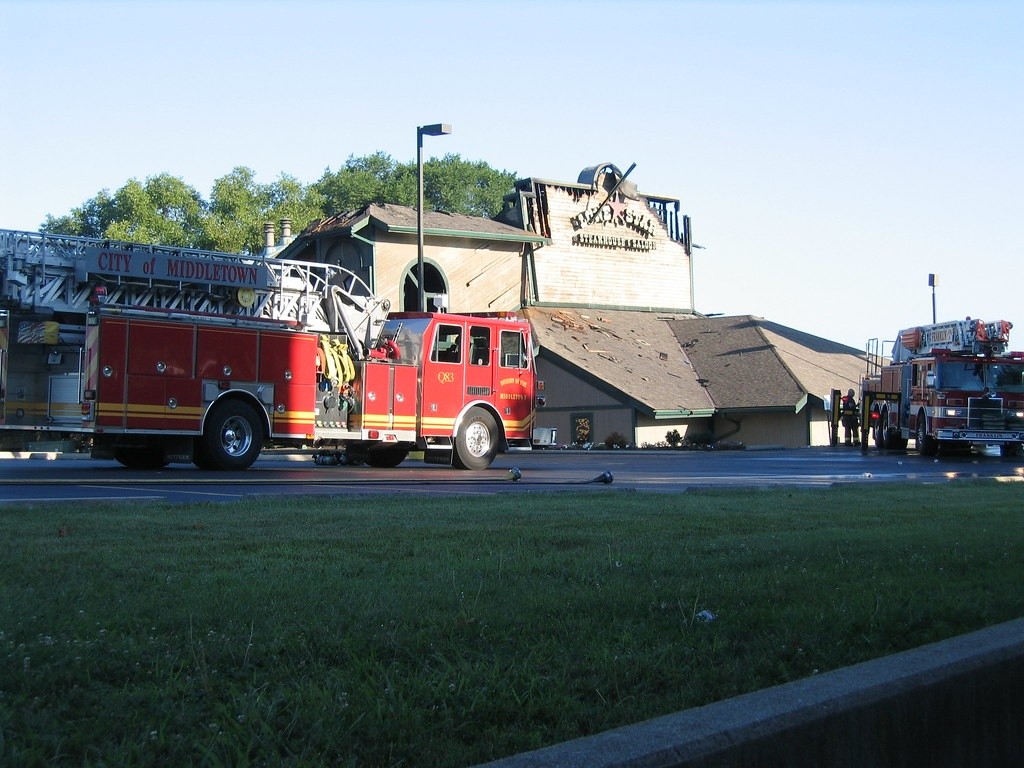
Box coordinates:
[856,315,1024,459]
[0,226,547,472]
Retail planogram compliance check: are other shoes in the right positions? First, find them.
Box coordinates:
[845,442,853,447]
[854,442,861,446]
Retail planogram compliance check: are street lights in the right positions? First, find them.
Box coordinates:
[927,273,939,326]
[416,123,454,313]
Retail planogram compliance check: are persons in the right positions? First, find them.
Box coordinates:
[842,389,861,447]
